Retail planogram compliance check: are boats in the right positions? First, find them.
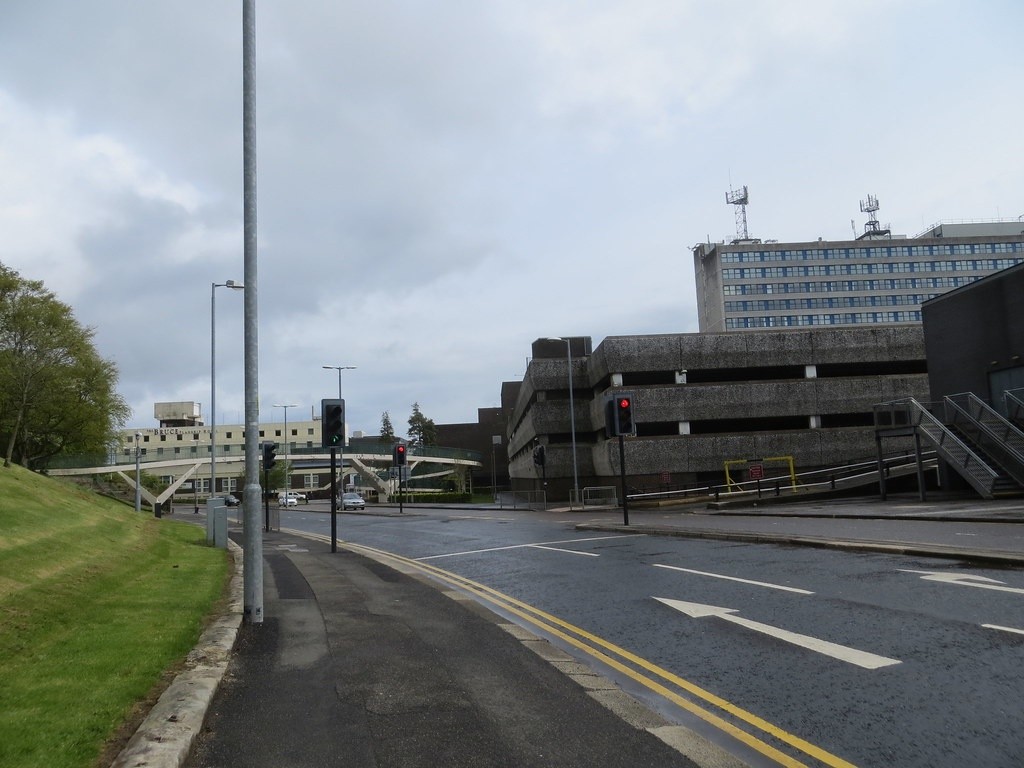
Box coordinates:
[133,433,142,514]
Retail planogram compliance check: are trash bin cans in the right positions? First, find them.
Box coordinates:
[161,498,171,513]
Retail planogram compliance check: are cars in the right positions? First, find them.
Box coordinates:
[284,492,306,501]
[337,491,365,511]
[218,495,240,507]
[279,495,299,506]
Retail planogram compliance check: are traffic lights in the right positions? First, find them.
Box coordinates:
[262,439,279,470]
[396,443,406,467]
[322,399,347,448]
[614,393,635,436]
[534,448,545,464]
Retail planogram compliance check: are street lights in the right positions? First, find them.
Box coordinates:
[545,336,579,506]
[209,279,246,494]
[322,364,356,514]
[273,403,300,511]
